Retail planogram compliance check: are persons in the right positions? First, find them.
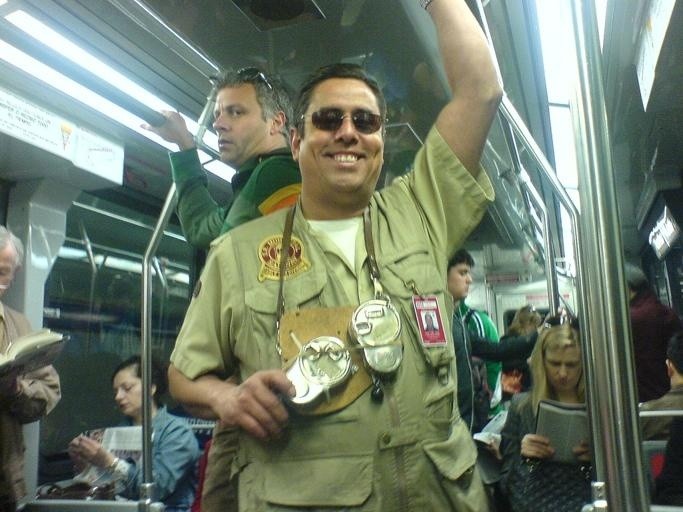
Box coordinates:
[168,0,504,512]
[68,353,199,511]
[139,63,303,512]
[421,309,441,339]
[447,248,683,511]
[0,225,61,512]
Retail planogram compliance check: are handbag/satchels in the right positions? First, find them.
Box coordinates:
[506,456,593,510]
[36,477,116,500]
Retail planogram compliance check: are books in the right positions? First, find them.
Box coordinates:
[0,329,70,385]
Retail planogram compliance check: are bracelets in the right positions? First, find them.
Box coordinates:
[418,0,439,8]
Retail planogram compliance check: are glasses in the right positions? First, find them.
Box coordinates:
[302,110,388,134]
[231,66,272,90]
